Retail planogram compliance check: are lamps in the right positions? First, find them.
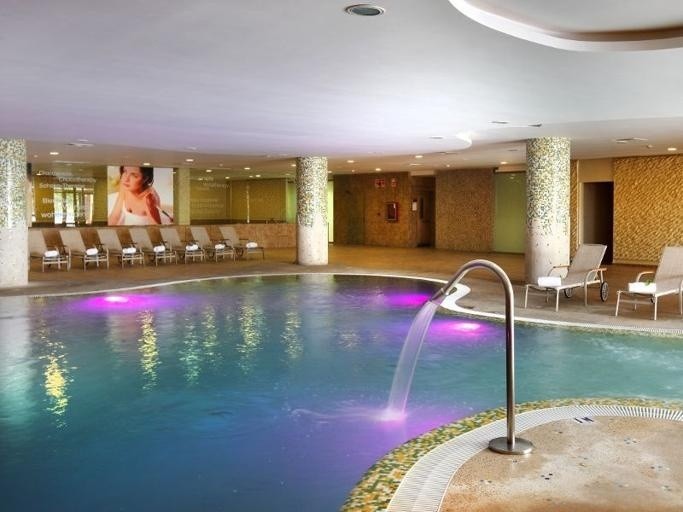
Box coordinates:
[346,4,384,17]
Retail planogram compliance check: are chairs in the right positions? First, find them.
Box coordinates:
[523,243,609,311]
[615,247,682,320]
[29,226,266,272]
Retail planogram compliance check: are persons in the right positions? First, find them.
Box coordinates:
[107,165,161,226]
